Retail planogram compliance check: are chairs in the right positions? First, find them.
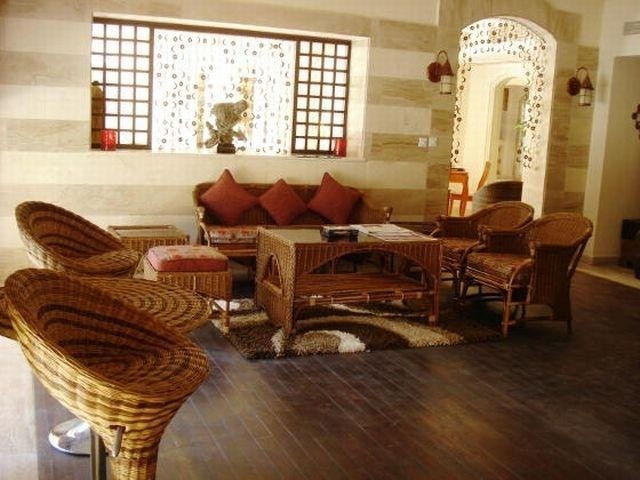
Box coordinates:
[15,201,141,277]
[459,213,593,338]
[3,268,211,480]
[472,182,522,215]
[449,162,493,218]
[429,200,534,302]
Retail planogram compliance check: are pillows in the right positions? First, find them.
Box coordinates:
[199,169,257,227]
[257,179,308,226]
[306,172,365,225]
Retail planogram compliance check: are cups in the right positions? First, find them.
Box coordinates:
[333,139,347,156]
[99,129,116,151]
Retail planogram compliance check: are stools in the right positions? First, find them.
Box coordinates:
[392,220,439,285]
[133,245,233,337]
[108,225,191,255]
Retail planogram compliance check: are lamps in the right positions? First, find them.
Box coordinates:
[565,66,594,105]
[428,49,452,96]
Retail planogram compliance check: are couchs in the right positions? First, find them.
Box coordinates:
[194,183,393,257]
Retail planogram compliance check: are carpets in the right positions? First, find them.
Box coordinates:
[208,288,503,361]
